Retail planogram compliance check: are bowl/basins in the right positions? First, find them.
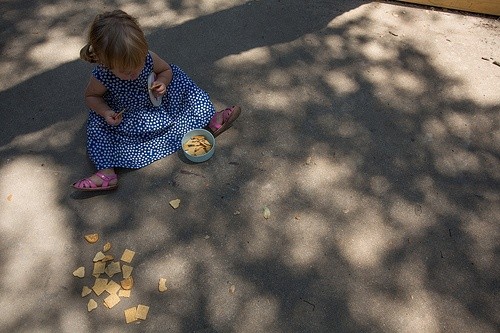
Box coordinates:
[182,129,215,162]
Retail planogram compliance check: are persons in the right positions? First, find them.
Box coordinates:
[68,8,242,193]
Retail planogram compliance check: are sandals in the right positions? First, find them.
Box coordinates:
[206,105,242,138]
[70,172,118,191]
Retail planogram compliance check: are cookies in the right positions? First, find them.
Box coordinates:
[170,198,181,208]
[72,233,167,323]
[150,84,155,90]
[184,135,213,156]
[115,108,125,119]
